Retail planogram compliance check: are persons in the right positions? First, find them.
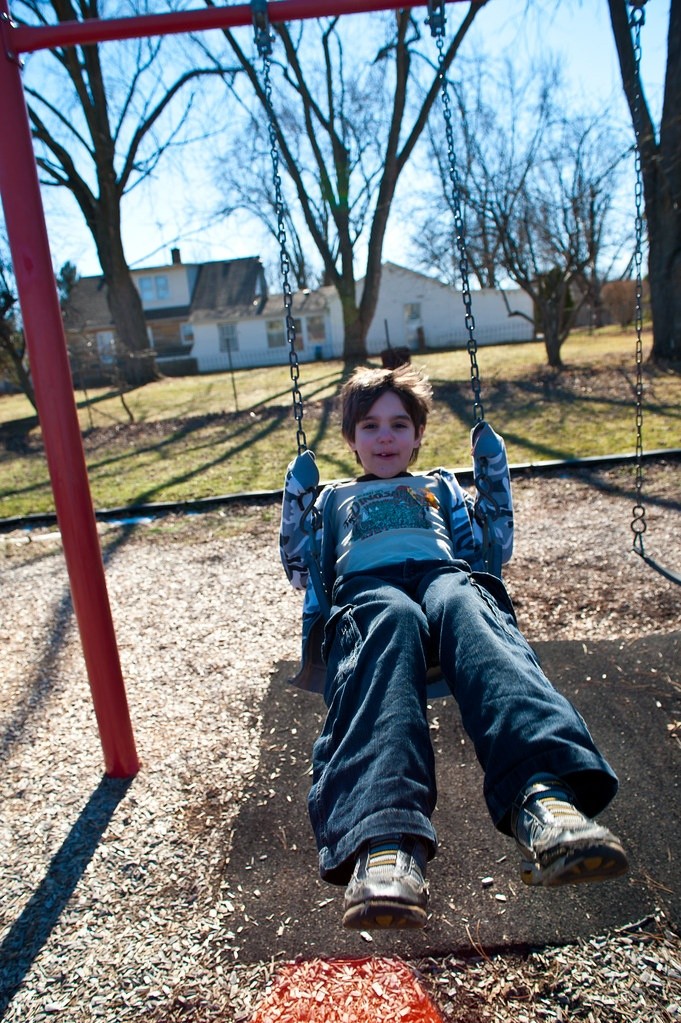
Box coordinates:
[276,364,629,929]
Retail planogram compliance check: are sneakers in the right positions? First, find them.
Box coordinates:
[510,778,628,887]
[343,833,429,929]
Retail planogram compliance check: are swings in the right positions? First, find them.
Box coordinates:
[628,0,681,585]
[246,0,519,700]
